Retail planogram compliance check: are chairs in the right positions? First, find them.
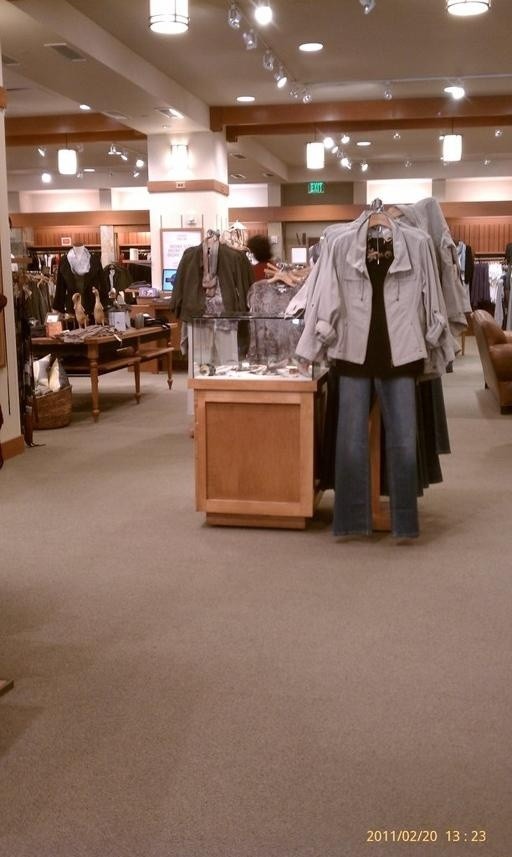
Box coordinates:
[470,308,511,415]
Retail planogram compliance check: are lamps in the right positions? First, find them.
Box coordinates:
[300,125,331,174]
[434,85,473,168]
[56,128,83,179]
[145,1,195,41]
[317,127,381,175]
[221,2,326,110]
[442,1,492,23]
[75,101,152,176]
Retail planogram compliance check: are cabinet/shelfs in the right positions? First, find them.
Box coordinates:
[188,312,329,531]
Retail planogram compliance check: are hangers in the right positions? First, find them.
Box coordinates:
[203,198,405,288]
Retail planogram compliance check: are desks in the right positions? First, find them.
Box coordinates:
[29,320,179,426]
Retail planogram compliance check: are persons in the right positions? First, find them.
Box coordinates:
[51,241,109,328]
[246,233,276,282]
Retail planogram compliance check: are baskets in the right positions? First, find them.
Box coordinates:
[34,384,73,431]
